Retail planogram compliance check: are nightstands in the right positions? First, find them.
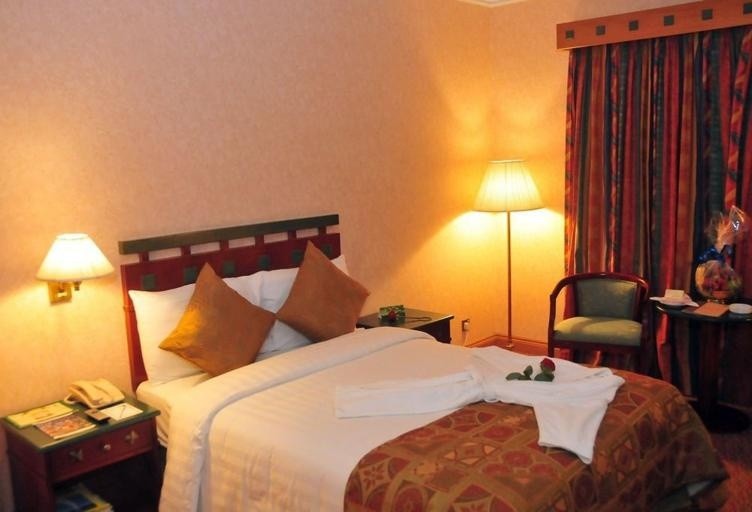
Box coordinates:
[0,393,161,512]
[356,307,454,345]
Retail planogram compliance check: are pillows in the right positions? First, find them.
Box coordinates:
[278,239,369,346]
[259,255,350,354]
[127,271,263,381]
[159,262,277,377]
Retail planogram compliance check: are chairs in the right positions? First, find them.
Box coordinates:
[547,271,651,375]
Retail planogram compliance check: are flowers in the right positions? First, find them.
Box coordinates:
[508,359,557,381]
[388,310,430,324]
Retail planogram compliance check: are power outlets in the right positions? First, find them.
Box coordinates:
[462,319,470,332]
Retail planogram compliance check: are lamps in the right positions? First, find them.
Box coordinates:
[35,233,114,305]
[470,160,545,351]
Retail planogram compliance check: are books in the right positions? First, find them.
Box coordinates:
[35,415,96,441]
[7,402,79,429]
[52,482,114,512]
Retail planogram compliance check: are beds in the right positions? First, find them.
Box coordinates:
[118,215,676,512]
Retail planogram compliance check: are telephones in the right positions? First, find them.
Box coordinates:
[69,377,125,409]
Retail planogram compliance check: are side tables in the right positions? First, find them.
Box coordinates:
[649,296,752,434]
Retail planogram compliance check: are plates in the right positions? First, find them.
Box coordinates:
[658,297,687,308]
[729,303,752,314]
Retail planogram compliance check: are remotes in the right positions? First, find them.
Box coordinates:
[84,409,111,424]
